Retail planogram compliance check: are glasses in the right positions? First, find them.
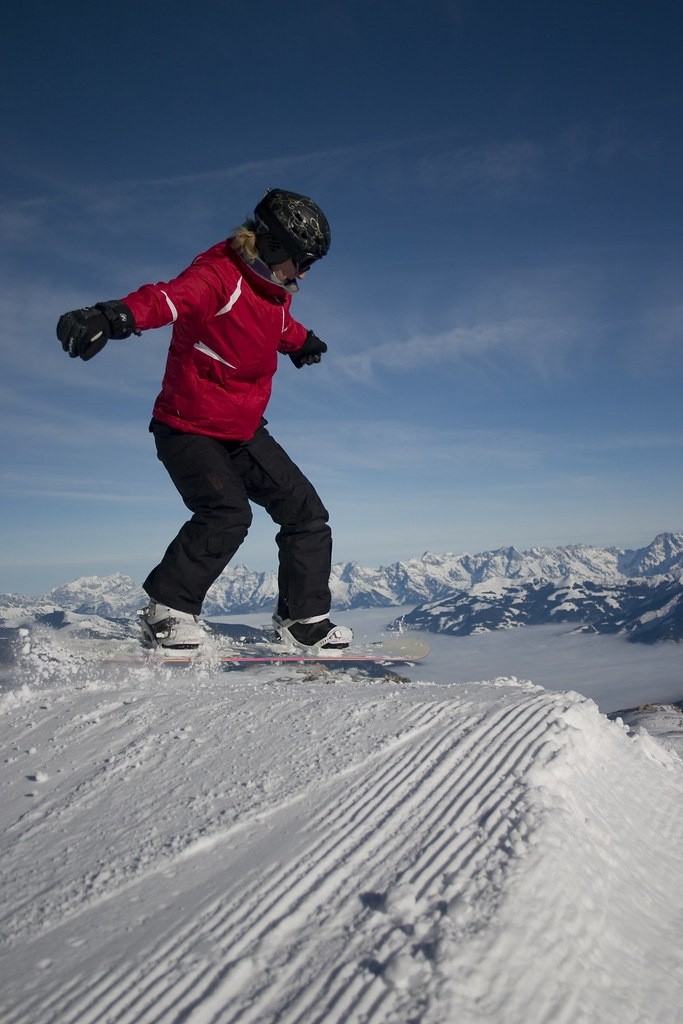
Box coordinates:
[292,254,311,274]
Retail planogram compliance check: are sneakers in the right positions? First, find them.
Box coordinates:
[139,596,205,658]
[271,605,354,658]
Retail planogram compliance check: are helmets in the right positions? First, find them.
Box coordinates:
[254,188,331,267]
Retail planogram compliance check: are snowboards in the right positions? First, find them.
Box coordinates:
[16,637,429,667]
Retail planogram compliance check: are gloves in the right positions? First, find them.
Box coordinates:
[56,300,142,361]
[289,330,328,370]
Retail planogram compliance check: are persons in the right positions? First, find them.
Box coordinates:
[57,190,352,657]
